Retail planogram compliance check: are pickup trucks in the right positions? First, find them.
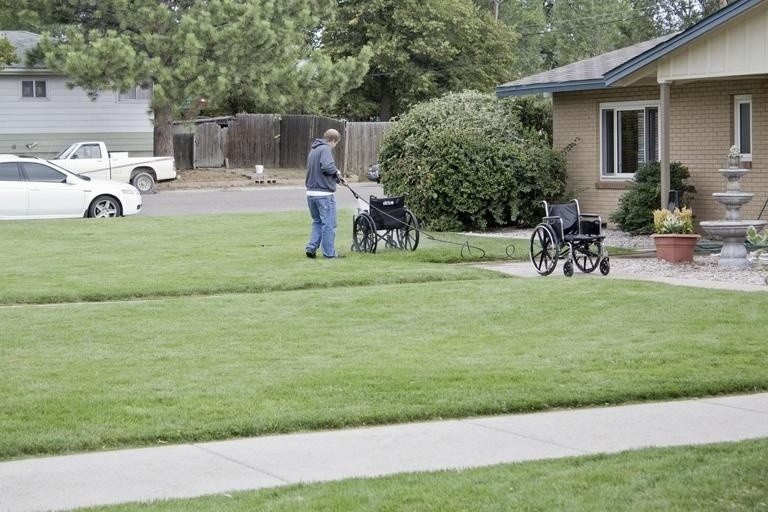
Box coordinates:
[42,142,177,194]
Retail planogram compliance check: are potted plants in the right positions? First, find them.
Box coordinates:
[649,204,701,264]
[744,224,768,254]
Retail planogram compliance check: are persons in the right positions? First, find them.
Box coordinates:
[304,127,347,260]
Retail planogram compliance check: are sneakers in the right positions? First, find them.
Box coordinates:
[306,252,316,258]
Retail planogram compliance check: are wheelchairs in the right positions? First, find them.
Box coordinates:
[528,197,612,279]
[351,190,419,257]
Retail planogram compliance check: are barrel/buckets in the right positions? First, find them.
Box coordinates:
[254,165,264,174]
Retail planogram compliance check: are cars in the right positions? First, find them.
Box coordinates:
[0,150,144,221]
[366,162,381,184]
